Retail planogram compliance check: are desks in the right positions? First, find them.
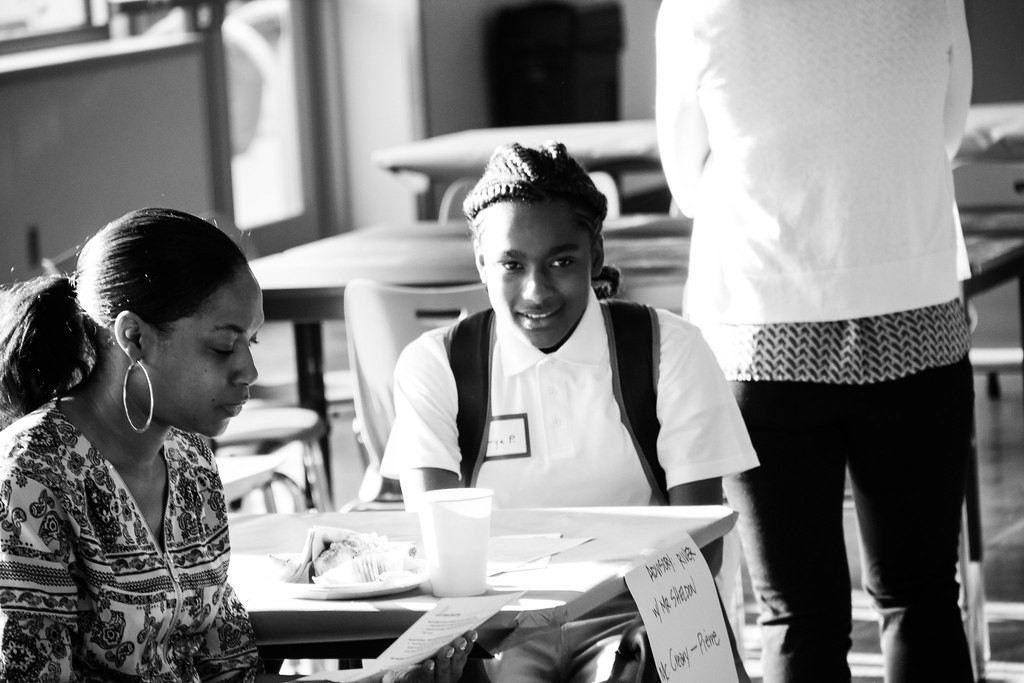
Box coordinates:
[372,102,1024,402]
[244,219,1024,683]
[224,504,740,683]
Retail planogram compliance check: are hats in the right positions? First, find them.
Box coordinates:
[463,141,607,239]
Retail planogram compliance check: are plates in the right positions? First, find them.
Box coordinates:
[286,579,425,599]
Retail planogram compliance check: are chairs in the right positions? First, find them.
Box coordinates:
[210,172,619,516]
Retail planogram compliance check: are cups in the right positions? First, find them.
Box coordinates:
[418,488,492,596]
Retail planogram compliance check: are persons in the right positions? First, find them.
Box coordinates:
[0,207,289,683]
[656,0,973,683]
[379,143,761,683]
